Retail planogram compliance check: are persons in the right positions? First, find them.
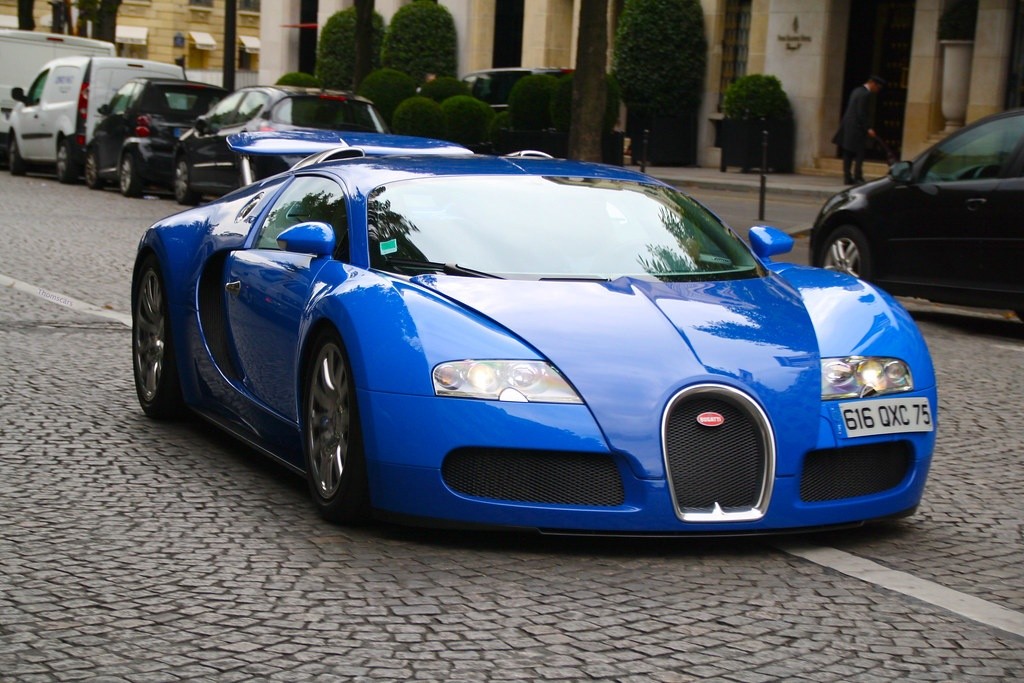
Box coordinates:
[841,76,881,185]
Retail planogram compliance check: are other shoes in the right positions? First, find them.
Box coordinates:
[844,176,866,185]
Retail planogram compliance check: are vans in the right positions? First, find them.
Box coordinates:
[0,29,117,169]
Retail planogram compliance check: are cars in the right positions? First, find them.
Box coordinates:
[7,55,186,185]
[458,64,626,133]
[83,75,232,197]
[173,84,394,208]
[810,106,1024,317]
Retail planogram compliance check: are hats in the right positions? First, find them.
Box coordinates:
[869,72,885,85]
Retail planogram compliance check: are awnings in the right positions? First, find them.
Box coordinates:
[114,25,149,45]
[239,36,261,53]
[187,32,218,51]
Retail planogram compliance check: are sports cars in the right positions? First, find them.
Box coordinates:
[132,128,941,543]
